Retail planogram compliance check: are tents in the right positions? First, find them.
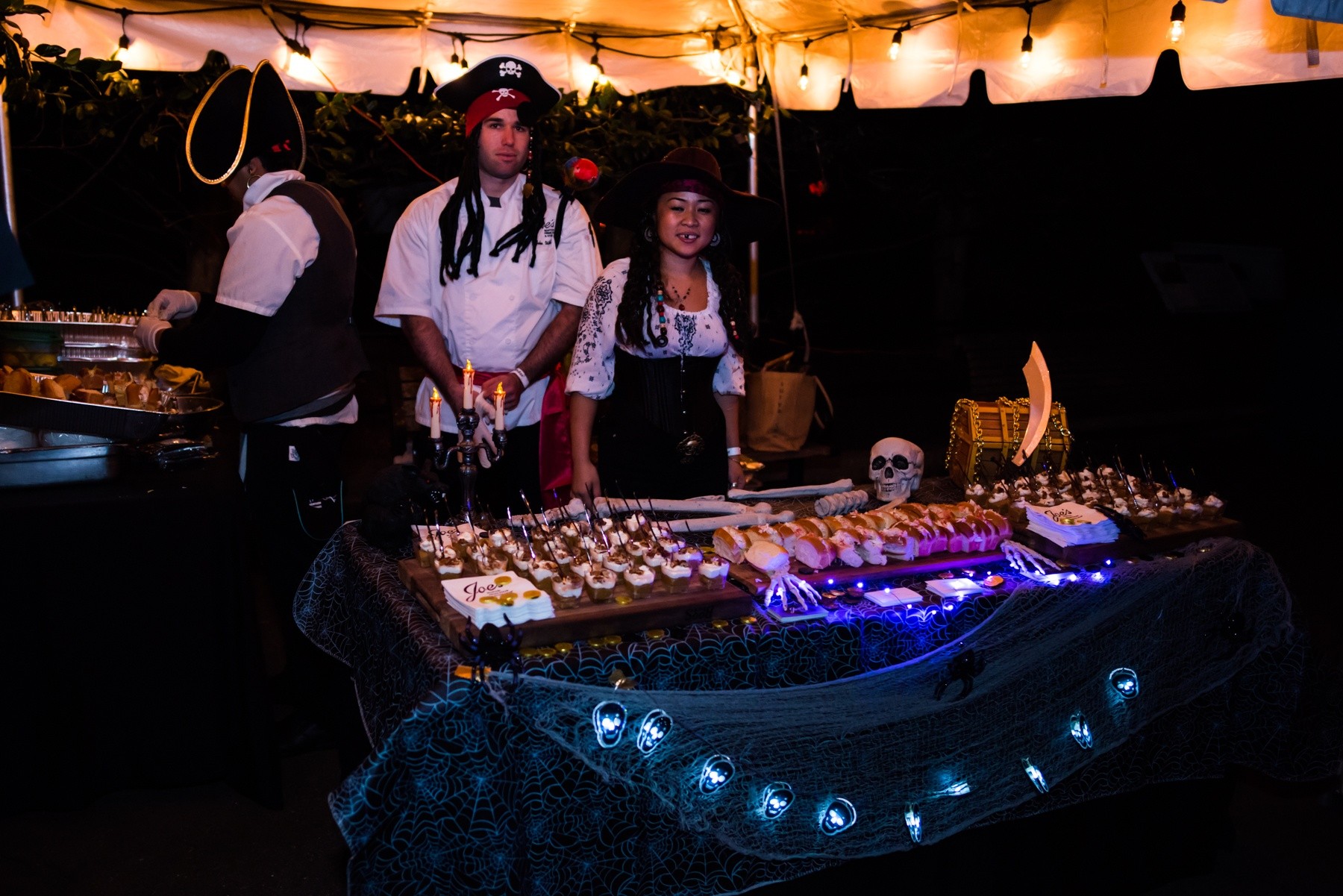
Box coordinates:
[0,0,1343,313]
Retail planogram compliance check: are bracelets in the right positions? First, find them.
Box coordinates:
[508,367,530,389]
[726,446,741,458]
[728,456,743,467]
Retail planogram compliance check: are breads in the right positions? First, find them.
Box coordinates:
[1,362,170,415]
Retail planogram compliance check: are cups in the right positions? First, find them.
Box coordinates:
[415,519,729,602]
[965,467,1223,531]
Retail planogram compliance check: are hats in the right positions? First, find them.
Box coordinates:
[593,146,782,239]
[435,54,562,286]
[184,59,307,186]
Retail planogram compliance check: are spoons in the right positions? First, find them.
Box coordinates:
[956,455,1217,514]
[406,481,710,580]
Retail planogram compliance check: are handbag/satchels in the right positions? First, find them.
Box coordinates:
[744,352,834,452]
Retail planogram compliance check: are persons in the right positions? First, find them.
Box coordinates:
[373,82,605,514]
[132,118,364,760]
[565,146,751,516]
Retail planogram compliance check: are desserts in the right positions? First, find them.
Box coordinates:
[409,484,729,629]
[712,500,1013,576]
[965,465,1225,529]
[0,302,144,325]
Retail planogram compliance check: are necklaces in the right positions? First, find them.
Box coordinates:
[663,259,695,310]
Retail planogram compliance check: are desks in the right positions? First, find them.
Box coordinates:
[338,450,1282,891]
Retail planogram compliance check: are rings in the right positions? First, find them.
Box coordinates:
[729,482,738,488]
